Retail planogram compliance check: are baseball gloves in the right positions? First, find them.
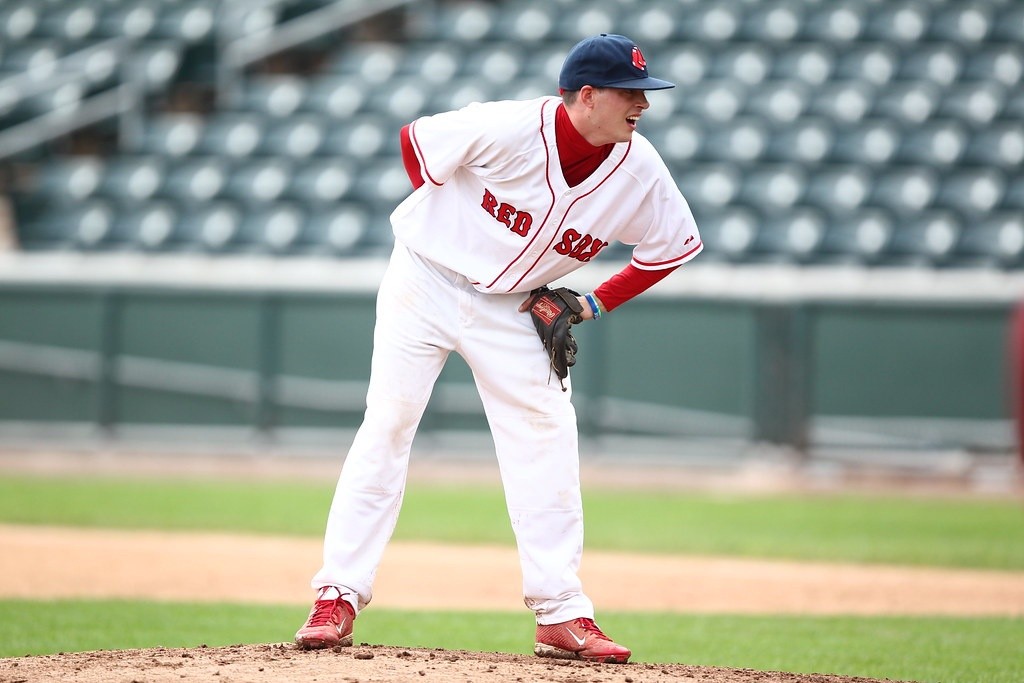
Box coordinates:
[528,283,584,379]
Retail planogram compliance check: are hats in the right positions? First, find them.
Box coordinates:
[559,33,675,90]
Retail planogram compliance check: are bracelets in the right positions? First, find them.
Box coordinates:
[585,292,602,320]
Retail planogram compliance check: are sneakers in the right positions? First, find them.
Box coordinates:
[293,587,355,648]
[535,617,631,664]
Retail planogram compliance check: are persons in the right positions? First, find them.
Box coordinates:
[292,33,705,663]
